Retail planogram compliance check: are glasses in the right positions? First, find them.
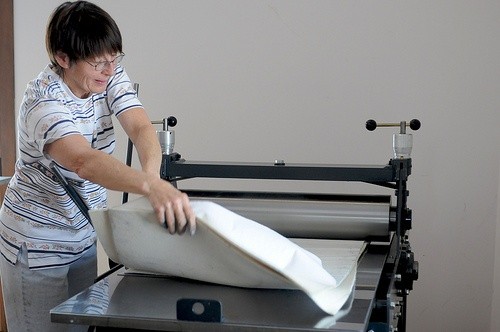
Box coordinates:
[78,51,126,71]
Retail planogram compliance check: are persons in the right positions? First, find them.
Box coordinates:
[0,0,195,332]
